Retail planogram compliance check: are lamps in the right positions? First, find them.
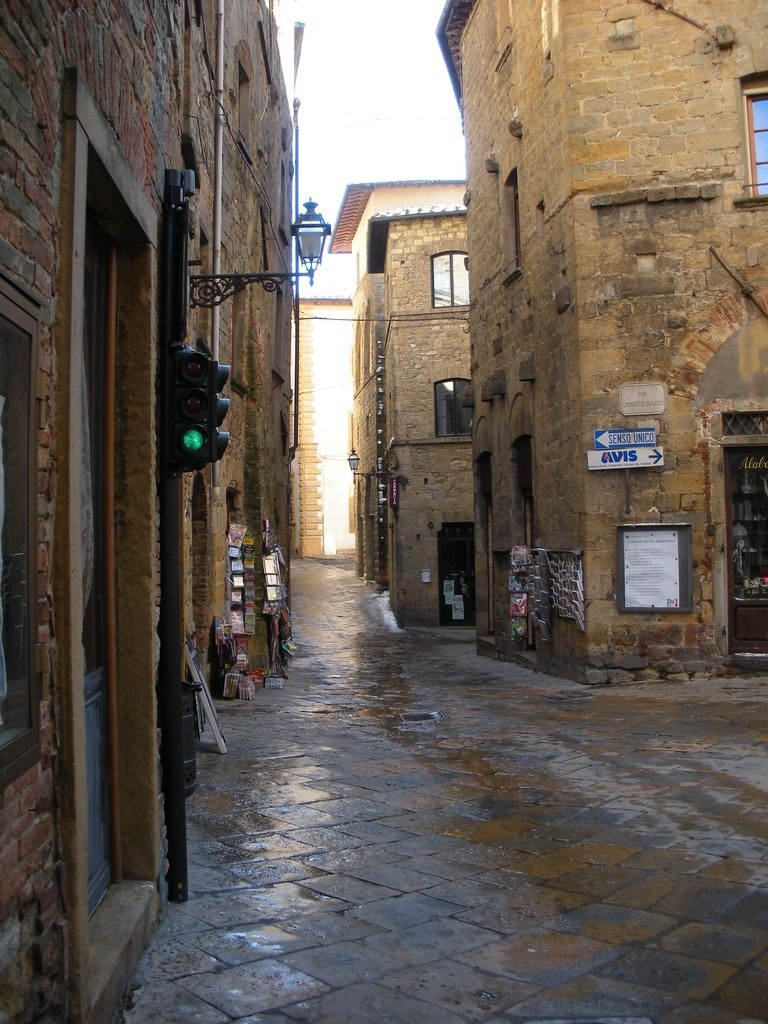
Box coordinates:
[347,447,377,484]
[189,196,332,310]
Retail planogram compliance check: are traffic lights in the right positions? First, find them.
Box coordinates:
[208,358,230,462]
[169,350,213,468]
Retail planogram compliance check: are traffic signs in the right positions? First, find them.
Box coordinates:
[594,427,657,450]
[587,446,664,470]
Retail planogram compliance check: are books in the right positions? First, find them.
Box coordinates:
[527,555,583,641]
[223,653,256,700]
